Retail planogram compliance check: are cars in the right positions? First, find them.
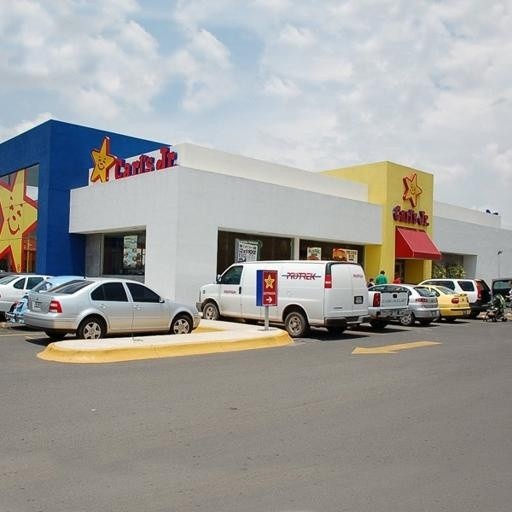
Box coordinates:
[367,278,490,326]
[0,273,51,313]
[25,277,193,343]
[6,275,84,323]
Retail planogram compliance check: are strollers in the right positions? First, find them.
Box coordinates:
[485,293,506,323]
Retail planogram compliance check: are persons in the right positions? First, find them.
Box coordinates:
[505,280,512,311]
[375,270,388,284]
[367,278,376,287]
[392,272,401,284]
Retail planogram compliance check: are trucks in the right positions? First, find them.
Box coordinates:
[195,259,367,338]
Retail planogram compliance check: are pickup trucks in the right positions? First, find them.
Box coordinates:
[368,287,409,334]
